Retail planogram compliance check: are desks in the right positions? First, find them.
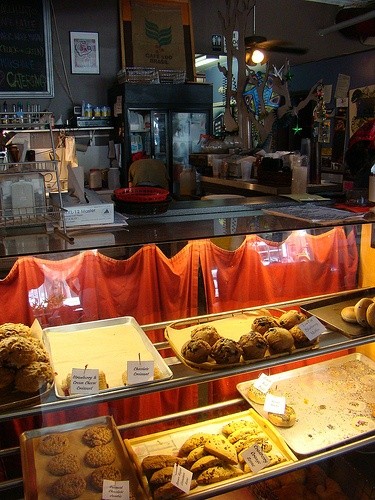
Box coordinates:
[198,176,338,195]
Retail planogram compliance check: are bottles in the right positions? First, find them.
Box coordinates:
[84,103,111,117]
[2,98,34,130]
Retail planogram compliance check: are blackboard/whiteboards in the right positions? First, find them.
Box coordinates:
[0,0,57,101]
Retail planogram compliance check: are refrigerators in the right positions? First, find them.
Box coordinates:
[106,75,215,202]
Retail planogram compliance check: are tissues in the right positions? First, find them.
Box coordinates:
[48,162,115,227]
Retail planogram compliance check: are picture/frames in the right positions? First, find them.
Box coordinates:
[69,31,100,75]
[119,0,197,84]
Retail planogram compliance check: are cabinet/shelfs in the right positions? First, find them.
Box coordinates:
[122,83,214,200]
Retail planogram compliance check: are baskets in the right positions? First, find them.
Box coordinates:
[116,66,155,85]
[154,69,186,84]
[113,186,169,202]
[110,185,172,215]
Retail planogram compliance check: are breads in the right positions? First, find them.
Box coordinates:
[142,380,297,500]
[0,322,58,395]
[41,425,137,500]
[340,295,375,328]
[182,309,318,364]
[62,353,161,401]
